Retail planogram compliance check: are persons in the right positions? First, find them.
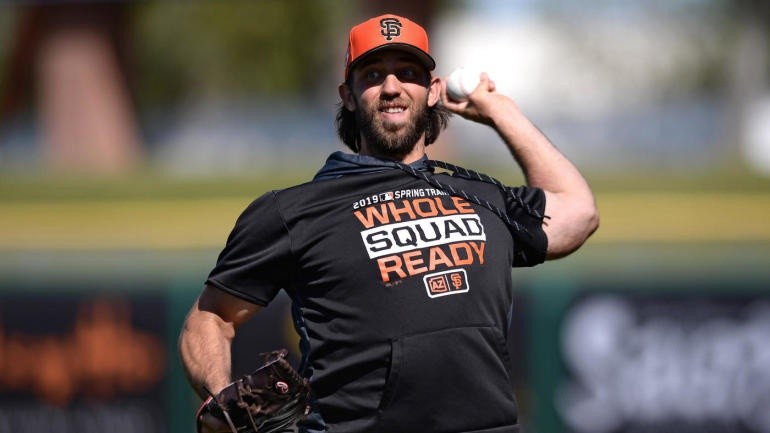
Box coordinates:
[179,13,600,433]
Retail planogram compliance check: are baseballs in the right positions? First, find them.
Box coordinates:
[446,67,481,103]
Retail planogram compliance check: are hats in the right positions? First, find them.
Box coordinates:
[344,13,435,80]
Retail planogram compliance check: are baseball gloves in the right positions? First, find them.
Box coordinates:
[195,347,315,433]
